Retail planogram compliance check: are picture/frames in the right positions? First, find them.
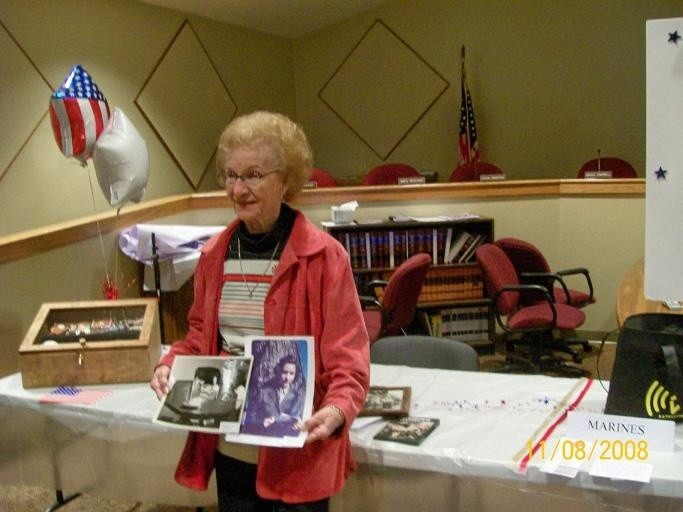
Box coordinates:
[357,386,410,418]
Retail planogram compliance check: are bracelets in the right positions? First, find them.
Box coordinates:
[327,402,346,421]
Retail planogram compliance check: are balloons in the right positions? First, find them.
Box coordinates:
[49,63,112,159]
[95,105,151,211]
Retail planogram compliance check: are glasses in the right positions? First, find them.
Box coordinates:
[219,169,279,184]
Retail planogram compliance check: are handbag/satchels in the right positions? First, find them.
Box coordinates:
[604,312,683,422]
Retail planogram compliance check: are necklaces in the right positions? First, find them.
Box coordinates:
[235,226,280,300]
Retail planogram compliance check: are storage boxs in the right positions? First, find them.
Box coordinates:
[19,297,162,387]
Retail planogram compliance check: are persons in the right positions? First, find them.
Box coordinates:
[249,353,304,437]
[150,108,371,512]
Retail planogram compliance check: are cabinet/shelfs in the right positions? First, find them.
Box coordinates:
[321,215,496,355]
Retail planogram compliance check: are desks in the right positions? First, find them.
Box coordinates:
[1,344,683,512]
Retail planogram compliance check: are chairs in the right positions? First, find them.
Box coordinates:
[359,238,596,378]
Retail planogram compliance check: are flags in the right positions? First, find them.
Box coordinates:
[39,386,112,406]
[457,45,481,166]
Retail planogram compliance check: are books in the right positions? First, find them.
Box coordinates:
[336,228,496,344]
